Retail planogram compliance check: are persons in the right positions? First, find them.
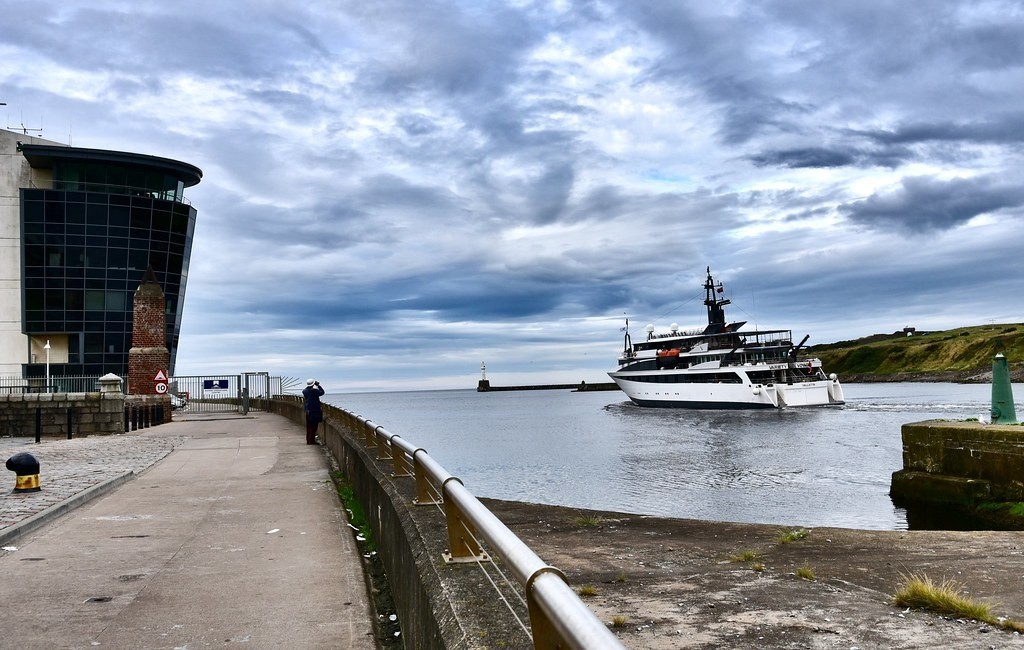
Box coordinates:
[302,379,325,445]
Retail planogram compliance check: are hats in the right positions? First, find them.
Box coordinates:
[304,378,316,387]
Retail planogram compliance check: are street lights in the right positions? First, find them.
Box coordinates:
[44,339,51,393]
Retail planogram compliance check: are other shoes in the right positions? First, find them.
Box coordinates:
[307,439,320,446]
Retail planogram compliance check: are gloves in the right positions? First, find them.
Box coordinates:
[314,381,320,386]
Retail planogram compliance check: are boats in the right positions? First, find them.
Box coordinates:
[606,265,847,409]
